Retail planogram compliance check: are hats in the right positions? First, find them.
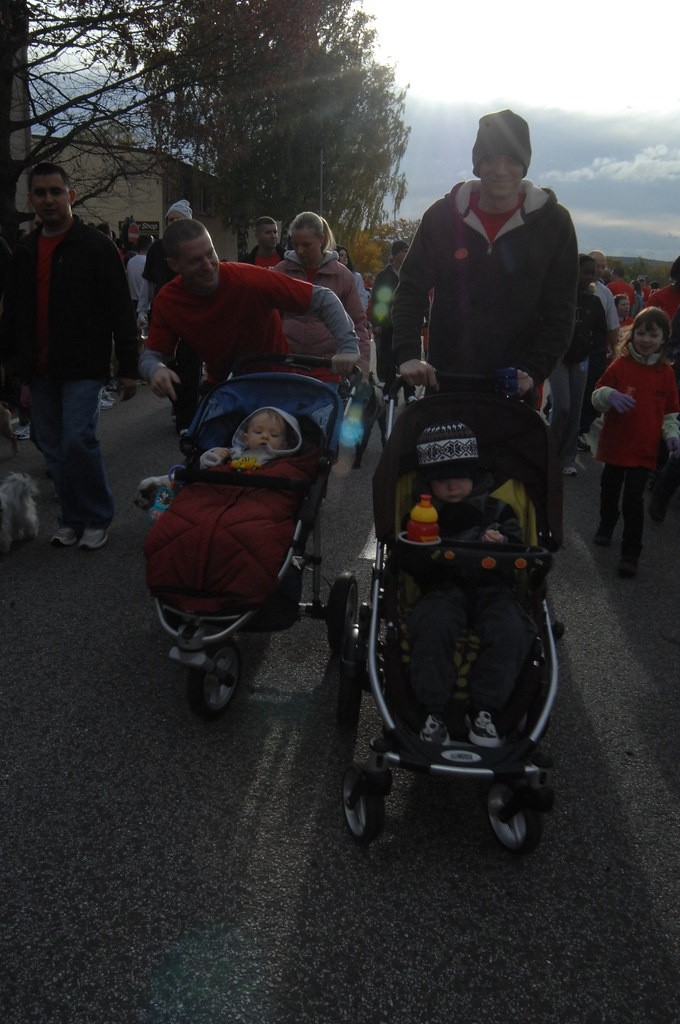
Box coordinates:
[165,199,193,220]
[415,415,480,469]
[473,114,531,179]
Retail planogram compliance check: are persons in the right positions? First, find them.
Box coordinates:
[0,200,680,577]
[9,163,159,551]
[392,109,580,635]
[146,407,309,616]
[396,414,536,749]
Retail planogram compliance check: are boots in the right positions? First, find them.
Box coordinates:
[594,509,621,548]
[616,541,643,577]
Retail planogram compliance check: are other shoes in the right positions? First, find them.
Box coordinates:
[13,425,31,439]
[648,494,671,523]
[100,390,117,409]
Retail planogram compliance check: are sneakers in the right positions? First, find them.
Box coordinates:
[576,435,591,451]
[561,467,577,475]
[77,529,108,550]
[463,711,500,748]
[50,527,79,546]
[419,714,451,746]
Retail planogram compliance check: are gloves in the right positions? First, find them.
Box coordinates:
[599,386,636,414]
[664,432,680,460]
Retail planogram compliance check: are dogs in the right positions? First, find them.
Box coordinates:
[133,473,176,512]
[0,472,41,552]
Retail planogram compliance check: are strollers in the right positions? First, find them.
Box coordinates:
[144,350,364,713]
[341,368,562,855]
